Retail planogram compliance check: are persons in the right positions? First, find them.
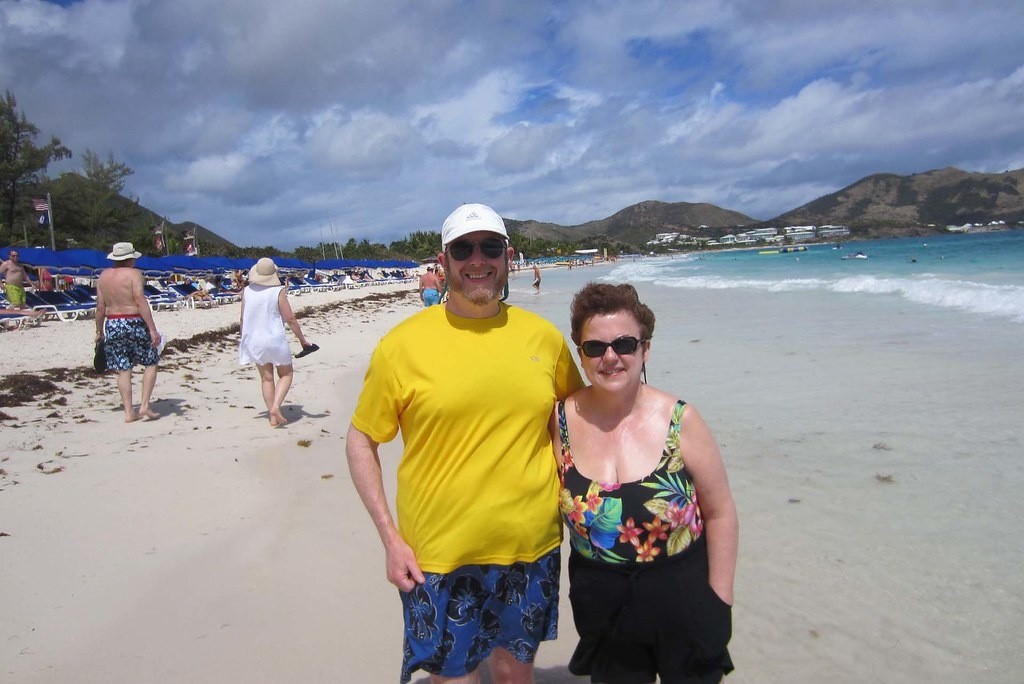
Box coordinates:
[239,257,313,427]
[40,268,53,292]
[418,264,442,307]
[94,241,162,425]
[65,276,74,291]
[548,282,739,684]
[532,264,542,295]
[185,259,573,310]
[0,251,35,310]
[345,202,583,684]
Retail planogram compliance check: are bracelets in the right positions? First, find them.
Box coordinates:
[97,330,102,334]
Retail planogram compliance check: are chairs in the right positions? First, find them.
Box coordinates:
[0,269,417,331]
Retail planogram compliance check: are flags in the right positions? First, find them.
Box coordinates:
[185,242,197,256]
[152,219,165,235]
[152,236,166,252]
[31,197,49,211]
[182,227,196,242]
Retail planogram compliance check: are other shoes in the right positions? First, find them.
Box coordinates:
[94,342,107,374]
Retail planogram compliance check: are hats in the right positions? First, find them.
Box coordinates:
[249,257,282,286]
[427,266,434,270]
[441,203,510,252]
[107,242,142,261]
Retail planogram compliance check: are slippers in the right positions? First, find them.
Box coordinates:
[295,343,319,359]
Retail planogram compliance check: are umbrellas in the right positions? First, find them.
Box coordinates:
[0,246,419,274]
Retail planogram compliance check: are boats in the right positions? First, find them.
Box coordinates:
[842,250,868,259]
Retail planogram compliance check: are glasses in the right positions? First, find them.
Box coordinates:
[11,255,19,257]
[577,336,645,357]
[444,238,506,261]
